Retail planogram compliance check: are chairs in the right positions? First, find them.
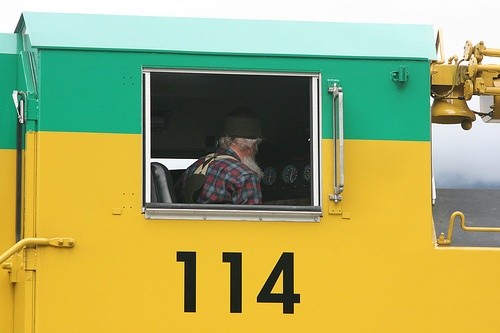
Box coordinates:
[150,162,181,204]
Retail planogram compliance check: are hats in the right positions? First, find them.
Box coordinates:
[221,106,263,139]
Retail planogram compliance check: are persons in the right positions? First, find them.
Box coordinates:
[179,113,266,204]
